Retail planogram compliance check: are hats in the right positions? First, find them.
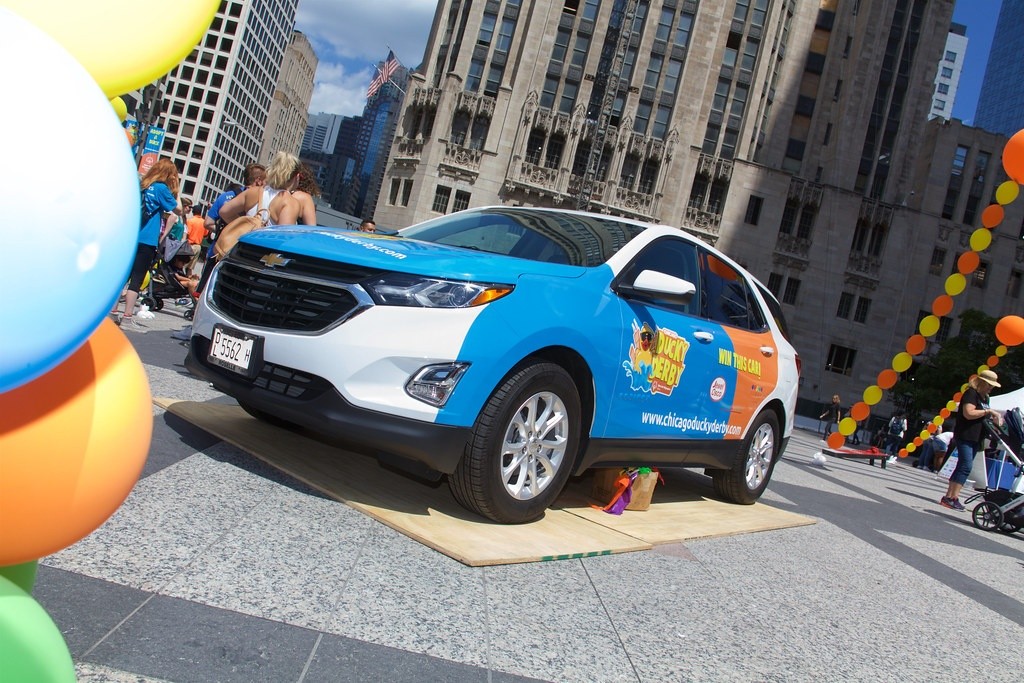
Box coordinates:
[977,370,1001,388]
[192,205,202,211]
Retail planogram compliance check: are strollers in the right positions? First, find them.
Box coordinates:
[140,237,197,321]
[964,407,1024,533]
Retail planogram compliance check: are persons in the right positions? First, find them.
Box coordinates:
[115,150,317,320]
[942,370,1002,512]
[360,220,376,234]
[851,412,867,446]
[820,394,841,444]
[874,409,954,475]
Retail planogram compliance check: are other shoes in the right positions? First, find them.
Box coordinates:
[917,466,923,469]
[820,440,825,442]
[851,441,859,445]
[934,470,938,473]
[923,466,929,471]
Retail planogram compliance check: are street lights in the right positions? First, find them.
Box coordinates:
[135,90,162,167]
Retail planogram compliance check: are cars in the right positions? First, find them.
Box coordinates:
[185,204,802,526]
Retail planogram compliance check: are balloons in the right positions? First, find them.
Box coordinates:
[0,576,78,683]
[1,316,151,572]
[0,0,226,100]
[0,30,139,397]
[827,130,1024,458]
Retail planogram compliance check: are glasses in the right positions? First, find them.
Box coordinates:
[187,205,192,208]
[368,228,375,232]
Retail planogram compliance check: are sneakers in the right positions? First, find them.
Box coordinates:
[107,312,150,334]
[941,496,966,512]
[170,325,192,340]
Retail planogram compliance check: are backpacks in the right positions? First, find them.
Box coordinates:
[211,186,270,262]
[890,416,906,434]
[1004,407,1024,462]
[139,207,162,232]
